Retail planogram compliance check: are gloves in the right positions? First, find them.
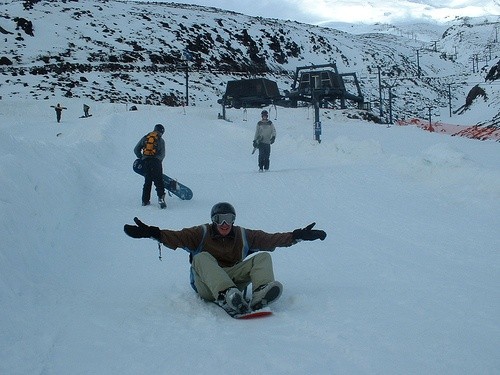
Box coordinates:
[124,217,160,241]
[270,136,275,144]
[293,222,326,243]
[253,140,257,147]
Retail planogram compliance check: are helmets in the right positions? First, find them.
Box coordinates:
[261,110,268,117]
[154,124,164,134]
[211,202,236,218]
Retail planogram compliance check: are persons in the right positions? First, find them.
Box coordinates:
[133,123,168,209]
[253,110,276,171]
[84,104,90,117]
[124,203,328,314]
[55,104,63,123]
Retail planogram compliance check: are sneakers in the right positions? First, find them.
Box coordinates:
[225,288,251,313]
[250,280,283,310]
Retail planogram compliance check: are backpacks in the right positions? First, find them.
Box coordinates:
[143,133,159,155]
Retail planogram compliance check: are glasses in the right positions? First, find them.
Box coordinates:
[212,213,235,225]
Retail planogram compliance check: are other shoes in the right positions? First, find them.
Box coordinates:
[259,169,262,173]
[264,168,269,172]
[142,201,150,206]
[158,193,166,208]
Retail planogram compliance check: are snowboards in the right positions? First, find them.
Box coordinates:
[132,158,194,200]
[213,295,273,319]
[252,135,264,154]
